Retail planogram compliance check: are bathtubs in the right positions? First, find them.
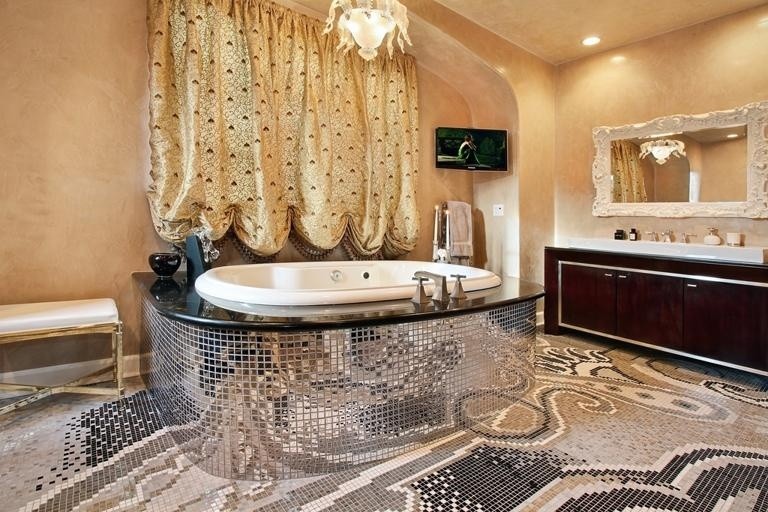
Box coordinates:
[192,259,502,307]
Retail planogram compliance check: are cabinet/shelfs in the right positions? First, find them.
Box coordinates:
[542,246,767,379]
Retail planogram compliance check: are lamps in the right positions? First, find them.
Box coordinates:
[320,0,413,64]
[634,135,688,166]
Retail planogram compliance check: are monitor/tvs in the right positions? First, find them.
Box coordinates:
[435,126,509,173]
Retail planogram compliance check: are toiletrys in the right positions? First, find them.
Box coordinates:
[629,228,638,241]
[614,229,625,240]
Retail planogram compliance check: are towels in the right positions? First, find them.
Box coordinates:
[447,200,474,258]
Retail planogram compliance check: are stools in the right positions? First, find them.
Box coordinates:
[0,296,126,417]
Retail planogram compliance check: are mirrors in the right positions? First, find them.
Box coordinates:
[590,99,767,222]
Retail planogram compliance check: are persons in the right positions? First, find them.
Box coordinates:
[457,133,481,166]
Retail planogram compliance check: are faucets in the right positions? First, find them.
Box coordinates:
[660,229,672,242]
[414,270,449,302]
